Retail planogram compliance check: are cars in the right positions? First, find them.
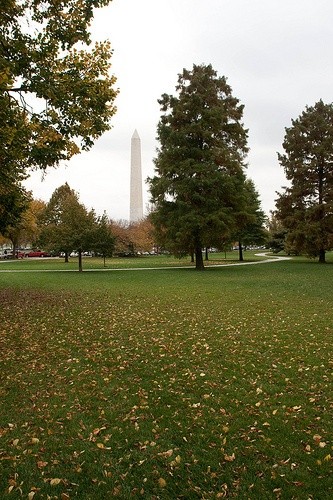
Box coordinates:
[0,249,88,259]
